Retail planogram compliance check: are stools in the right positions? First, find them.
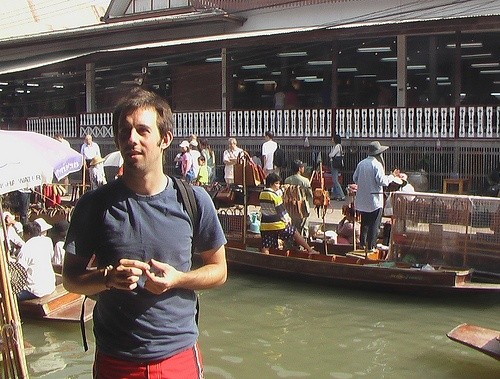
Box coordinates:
[442,178,472,194]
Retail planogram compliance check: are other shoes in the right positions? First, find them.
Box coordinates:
[330,196,346,201]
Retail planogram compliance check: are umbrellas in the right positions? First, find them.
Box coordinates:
[0,130,83,195]
[102,150,124,168]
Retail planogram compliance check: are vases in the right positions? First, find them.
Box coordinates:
[405,171,429,192]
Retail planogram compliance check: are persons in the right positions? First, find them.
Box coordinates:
[174,130,416,255]
[0,132,123,303]
[62,86,227,379]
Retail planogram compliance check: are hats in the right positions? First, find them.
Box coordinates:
[179,141,190,148]
[90,156,105,165]
[34,218,53,232]
[366,141,389,156]
[190,139,198,147]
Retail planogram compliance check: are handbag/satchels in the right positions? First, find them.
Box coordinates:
[281,184,311,220]
[313,188,330,206]
[234,151,266,187]
[332,146,343,169]
[383,192,394,215]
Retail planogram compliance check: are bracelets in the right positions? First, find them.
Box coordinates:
[103,265,112,290]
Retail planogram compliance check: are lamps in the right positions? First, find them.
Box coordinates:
[148,61,168,67]
[204,42,500,97]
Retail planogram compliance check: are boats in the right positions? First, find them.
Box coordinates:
[192,157,500,291]
[16,271,97,326]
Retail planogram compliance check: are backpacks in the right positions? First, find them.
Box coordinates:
[273,143,287,169]
